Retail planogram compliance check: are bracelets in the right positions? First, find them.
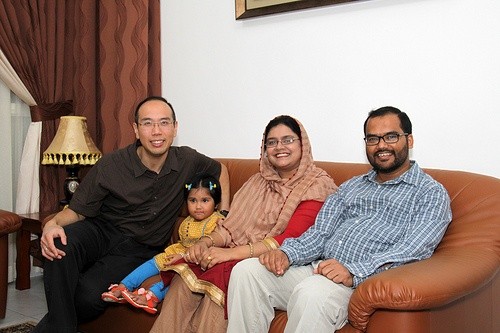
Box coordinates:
[247,242,253,258]
[199,235,215,248]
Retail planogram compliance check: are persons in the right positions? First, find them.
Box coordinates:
[102,172,225,314]
[29,96,229,333]
[227,106,453,333]
[149,115,338,333]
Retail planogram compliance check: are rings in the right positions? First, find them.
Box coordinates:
[44,248,48,251]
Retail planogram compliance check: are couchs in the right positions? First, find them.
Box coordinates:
[77,157,500,333]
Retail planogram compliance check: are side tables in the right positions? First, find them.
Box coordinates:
[14,209,61,291]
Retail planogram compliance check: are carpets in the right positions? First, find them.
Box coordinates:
[0,318,40,333]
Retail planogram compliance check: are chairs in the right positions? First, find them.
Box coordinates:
[0,207,24,321]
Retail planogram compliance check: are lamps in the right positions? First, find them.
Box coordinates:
[40,115,104,206]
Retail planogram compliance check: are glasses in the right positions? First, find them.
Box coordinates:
[364,133,409,146]
[135,120,175,128]
[265,137,300,147]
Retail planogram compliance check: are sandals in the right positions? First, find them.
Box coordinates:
[120,287,159,314]
[100,283,129,304]
